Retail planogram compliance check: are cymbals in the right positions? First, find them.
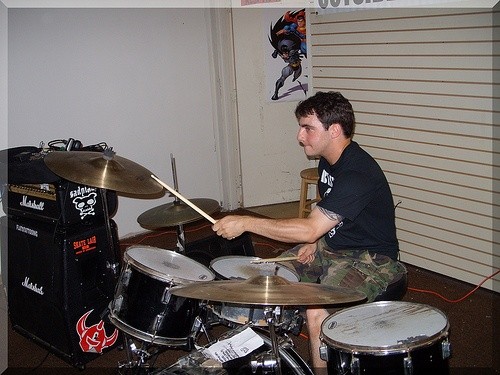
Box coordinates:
[168,275,368,306]
[43,151,164,195]
[137,199,221,230]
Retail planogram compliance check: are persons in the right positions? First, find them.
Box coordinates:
[211,91,404,375]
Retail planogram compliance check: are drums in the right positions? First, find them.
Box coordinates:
[318,300,452,375]
[155,321,315,375]
[198,256,302,331]
[106,244,216,348]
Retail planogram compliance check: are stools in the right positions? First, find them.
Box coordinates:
[298,167,321,218]
